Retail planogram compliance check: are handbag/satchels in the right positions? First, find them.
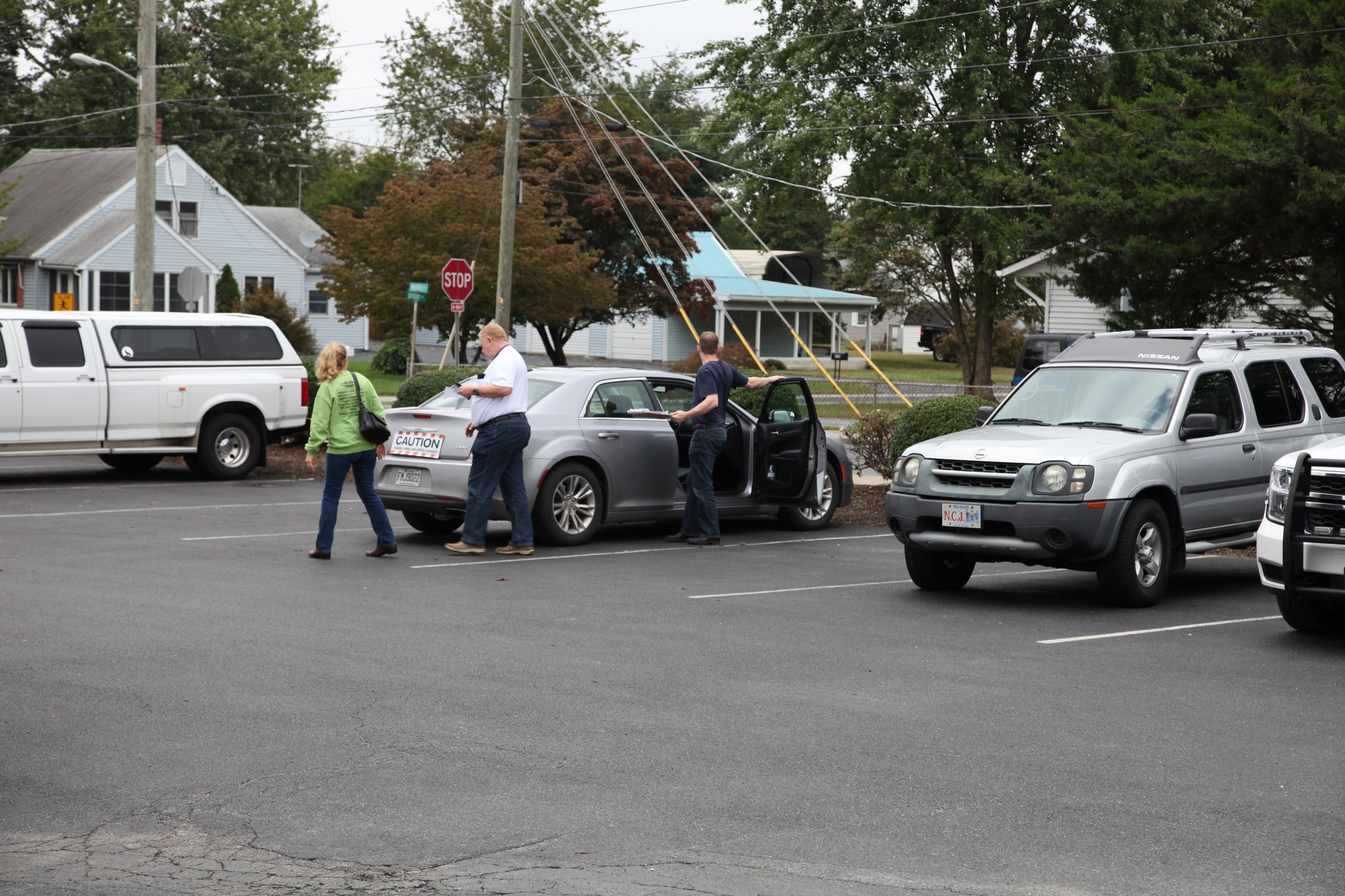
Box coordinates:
[349,371,392,446]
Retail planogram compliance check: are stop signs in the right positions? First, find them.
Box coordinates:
[441,258,475,302]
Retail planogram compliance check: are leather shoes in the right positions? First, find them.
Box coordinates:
[309,549,331,559]
[444,540,486,553]
[666,533,699,542]
[366,544,397,557]
[689,536,720,545]
[496,545,535,555]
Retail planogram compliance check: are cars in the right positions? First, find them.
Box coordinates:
[375,365,855,547]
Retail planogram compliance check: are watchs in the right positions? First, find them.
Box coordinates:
[473,386,479,397]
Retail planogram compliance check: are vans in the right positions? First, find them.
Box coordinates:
[0,308,310,481]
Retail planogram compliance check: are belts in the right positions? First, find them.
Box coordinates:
[695,424,725,430]
[476,412,525,430]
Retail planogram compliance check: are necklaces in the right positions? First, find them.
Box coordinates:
[492,344,510,360]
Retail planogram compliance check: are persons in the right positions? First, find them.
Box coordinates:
[304,343,397,559]
[444,323,534,555]
[664,330,787,545]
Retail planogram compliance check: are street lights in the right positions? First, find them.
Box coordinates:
[69,52,156,312]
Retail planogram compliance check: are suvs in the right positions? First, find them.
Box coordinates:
[1010,333,1090,388]
[885,327,1345,610]
[1255,432,1345,639]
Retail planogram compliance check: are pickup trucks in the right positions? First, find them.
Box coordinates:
[917,321,973,363]
